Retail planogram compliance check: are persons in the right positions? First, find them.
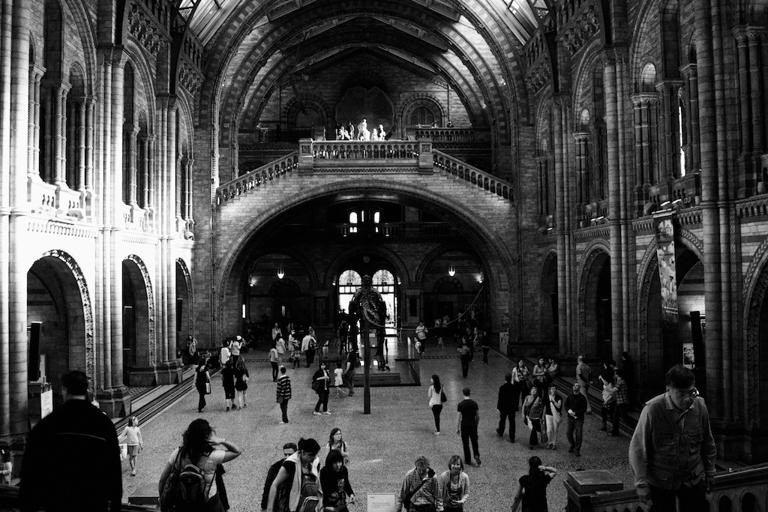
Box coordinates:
[437,455,470,512]
[0,447,13,486]
[117,416,144,476]
[628,365,716,512]
[394,455,444,512]
[414,310,491,379]
[16,369,123,511]
[456,388,481,466]
[261,428,356,512]
[495,351,631,457]
[510,456,558,512]
[335,118,387,142]
[180,322,356,425]
[428,375,445,437]
[157,418,242,511]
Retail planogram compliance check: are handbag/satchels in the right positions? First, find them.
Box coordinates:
[441,391,447,402]
[161,446,215,512]
[206,383,210,393]
[277,397,283,403]
